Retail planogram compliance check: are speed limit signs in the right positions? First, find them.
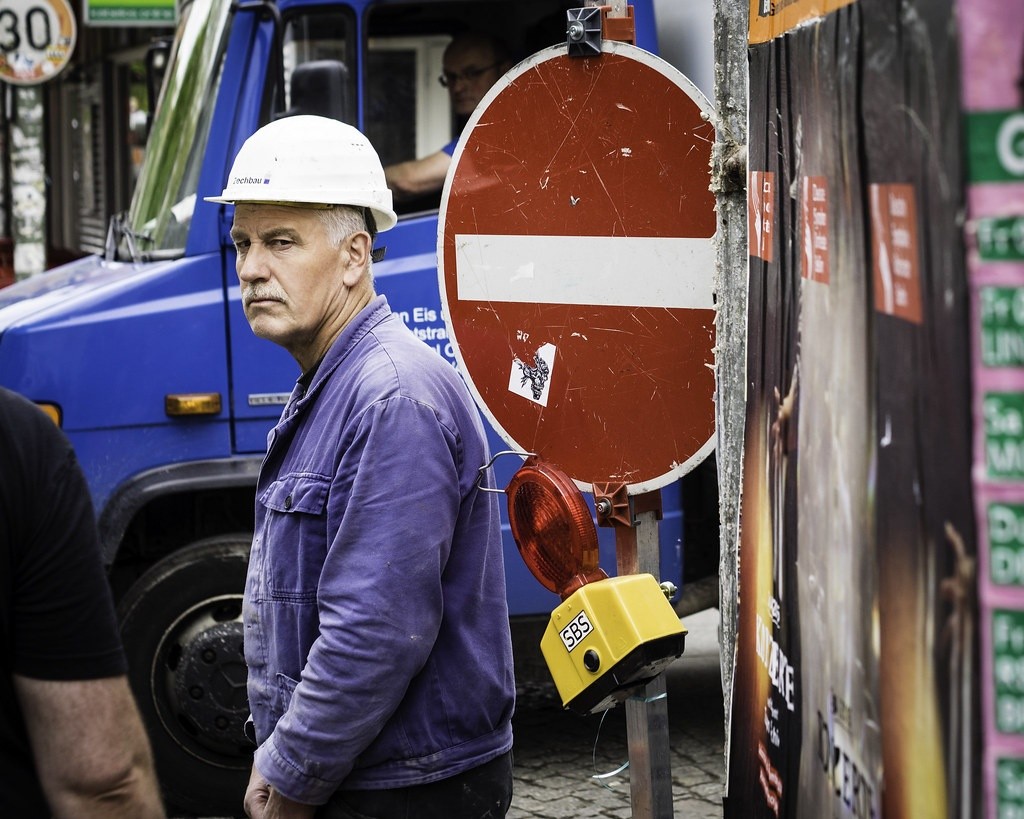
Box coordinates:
[0,0,77,86]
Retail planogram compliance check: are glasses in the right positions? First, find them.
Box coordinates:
[438,61,501,87]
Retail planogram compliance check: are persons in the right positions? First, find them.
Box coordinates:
[0,386,167,818]
[386,32,513,203]
[202,114,516,819]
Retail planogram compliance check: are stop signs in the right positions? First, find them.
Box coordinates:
[436,38,715,496]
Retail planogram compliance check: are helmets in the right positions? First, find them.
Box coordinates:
[201,114,396,234]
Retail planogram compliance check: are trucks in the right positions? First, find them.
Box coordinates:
[0,0,719,819]
